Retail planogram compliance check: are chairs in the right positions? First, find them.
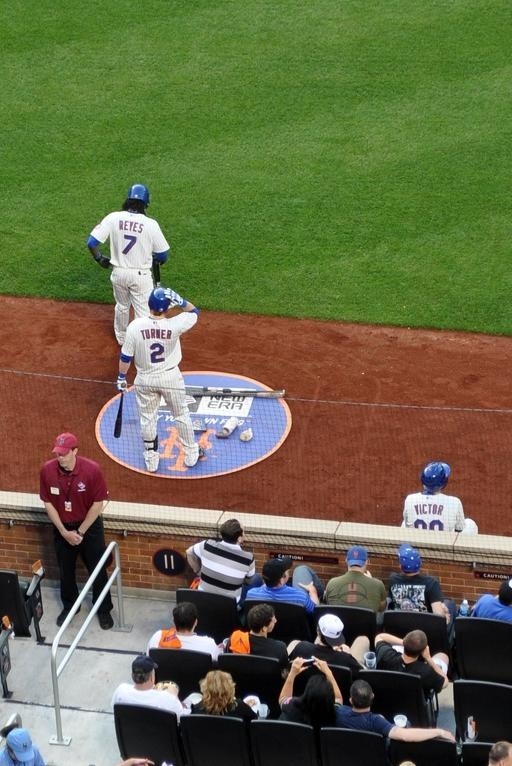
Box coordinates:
[0,566,47,699]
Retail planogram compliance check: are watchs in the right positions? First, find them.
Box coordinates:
[76,530,85,537]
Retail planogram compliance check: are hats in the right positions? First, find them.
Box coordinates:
[262,556,293,578]
[398,543,422,572]
[131,655,158,674]
[6,727,36,763]
[317,613,346,647]
[346,545,367,568]
[51,432,79,454]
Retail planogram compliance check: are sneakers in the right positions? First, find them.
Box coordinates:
[197,448,206,462]
[56,604,81,627]
[97,609,114,630]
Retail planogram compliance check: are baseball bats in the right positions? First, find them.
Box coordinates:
[152,257,161,286]
[114,382,126,437]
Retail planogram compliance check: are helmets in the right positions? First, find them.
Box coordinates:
[421,461,451,492]
[148,286,170,312]
[127,183,150,209]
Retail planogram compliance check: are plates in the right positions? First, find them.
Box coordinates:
[154,680,179,696]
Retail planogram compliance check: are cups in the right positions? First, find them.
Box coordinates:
[223,638,229,647]
[259,704,268,717]
[364,652,376,670]
[392,715,406,728]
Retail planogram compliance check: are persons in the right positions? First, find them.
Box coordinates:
[88,183,171,348]
[113,519,512,766]
[38,432,114,630]
[400,462,478,534]
[1,728,46,766]
[118,288,201,472]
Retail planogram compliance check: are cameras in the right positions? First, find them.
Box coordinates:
[301,658,314,668]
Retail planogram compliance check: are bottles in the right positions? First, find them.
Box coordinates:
[458,600,470,617]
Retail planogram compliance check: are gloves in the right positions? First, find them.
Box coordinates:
[163,287,185,310]
[94,251,112,268]
[116,375,129,393]
